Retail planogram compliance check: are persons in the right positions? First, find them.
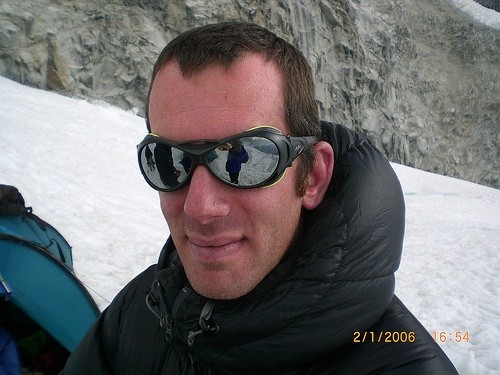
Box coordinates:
[226,137,249,183]
[154,142,181,188]
[144,144,156,171]
[54,19,461,375]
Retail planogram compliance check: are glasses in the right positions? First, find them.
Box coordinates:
[137,126,321,193]
